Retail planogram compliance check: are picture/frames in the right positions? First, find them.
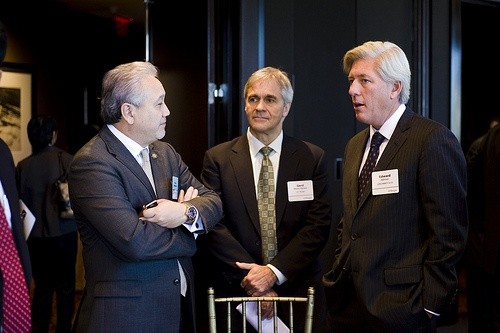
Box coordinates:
[0,62,39,164]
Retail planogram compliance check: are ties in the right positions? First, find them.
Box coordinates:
[0,202,32,333]
[357,131,385,207]
[141,148,188,296]
[257,146,277,266]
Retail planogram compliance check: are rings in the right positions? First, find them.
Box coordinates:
[248,290,253,295]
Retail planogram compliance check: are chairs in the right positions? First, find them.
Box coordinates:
[206,286,314,333]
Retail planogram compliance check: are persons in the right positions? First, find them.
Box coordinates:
[14,113,78,333]
[68,62,223,333]
[463,120,500,333]
[200,66,333,333]
[0,138,35,333]
[314,40,468,333]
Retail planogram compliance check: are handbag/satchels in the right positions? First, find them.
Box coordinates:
[55,151,76,219]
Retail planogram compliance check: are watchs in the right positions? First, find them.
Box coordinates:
[181,201,197,226]
